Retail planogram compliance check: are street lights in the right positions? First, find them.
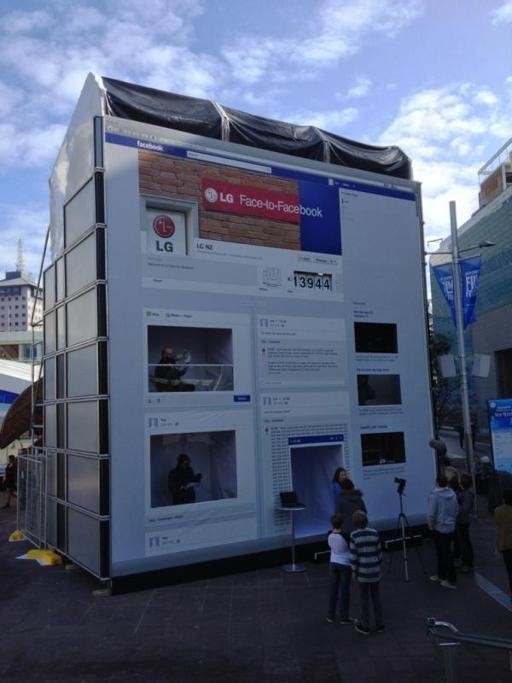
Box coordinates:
[425,198,496,528]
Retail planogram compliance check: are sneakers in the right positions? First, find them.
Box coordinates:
[376,626,386,633]
[326,616,336,624]
[341,617,359,625]
[354,624,371,636]
[428,557,474,590]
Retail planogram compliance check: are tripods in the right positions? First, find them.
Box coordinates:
[388,495,421,582]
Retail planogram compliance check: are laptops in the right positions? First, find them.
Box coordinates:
[280,492,306,508]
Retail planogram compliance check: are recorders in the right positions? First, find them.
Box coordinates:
[177,348,191,363]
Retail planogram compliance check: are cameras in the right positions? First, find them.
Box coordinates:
[394,477,406,494]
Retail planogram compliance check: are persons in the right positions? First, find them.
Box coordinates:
[333,477,368,541]
[444,465,460,560]
[441,466,477,567]
[168,452,204,505]
[329,466,348,514]
[348,507,387,637]
[491,486,511,594]
[153,347,194,392]
[325,512,355,624]
[427,471,458,589]
[1,454,20,510]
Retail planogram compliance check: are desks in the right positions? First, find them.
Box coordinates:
[275,504,310,574]
